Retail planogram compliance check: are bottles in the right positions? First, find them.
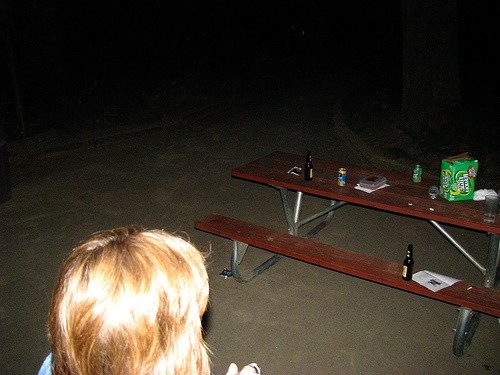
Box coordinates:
[402,243,414,281]
[304,150,313,180]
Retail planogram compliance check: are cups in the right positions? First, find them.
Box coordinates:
[483,195,499,223]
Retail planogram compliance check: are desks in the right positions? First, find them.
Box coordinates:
[231,152,500,357]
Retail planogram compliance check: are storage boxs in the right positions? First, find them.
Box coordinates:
[359,175,386,189]
[440,152,479,202]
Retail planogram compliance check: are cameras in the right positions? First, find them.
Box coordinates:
[359,174,386,189]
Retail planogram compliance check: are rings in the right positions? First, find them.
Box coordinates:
[249,365,258,373]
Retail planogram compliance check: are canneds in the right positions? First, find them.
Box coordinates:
[220,269,232,280]
[337,168,346,186]
[412,165,422,183]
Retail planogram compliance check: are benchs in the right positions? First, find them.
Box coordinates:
[194,213,500,321]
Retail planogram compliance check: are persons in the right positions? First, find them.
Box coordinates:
[37,223,261,375]
